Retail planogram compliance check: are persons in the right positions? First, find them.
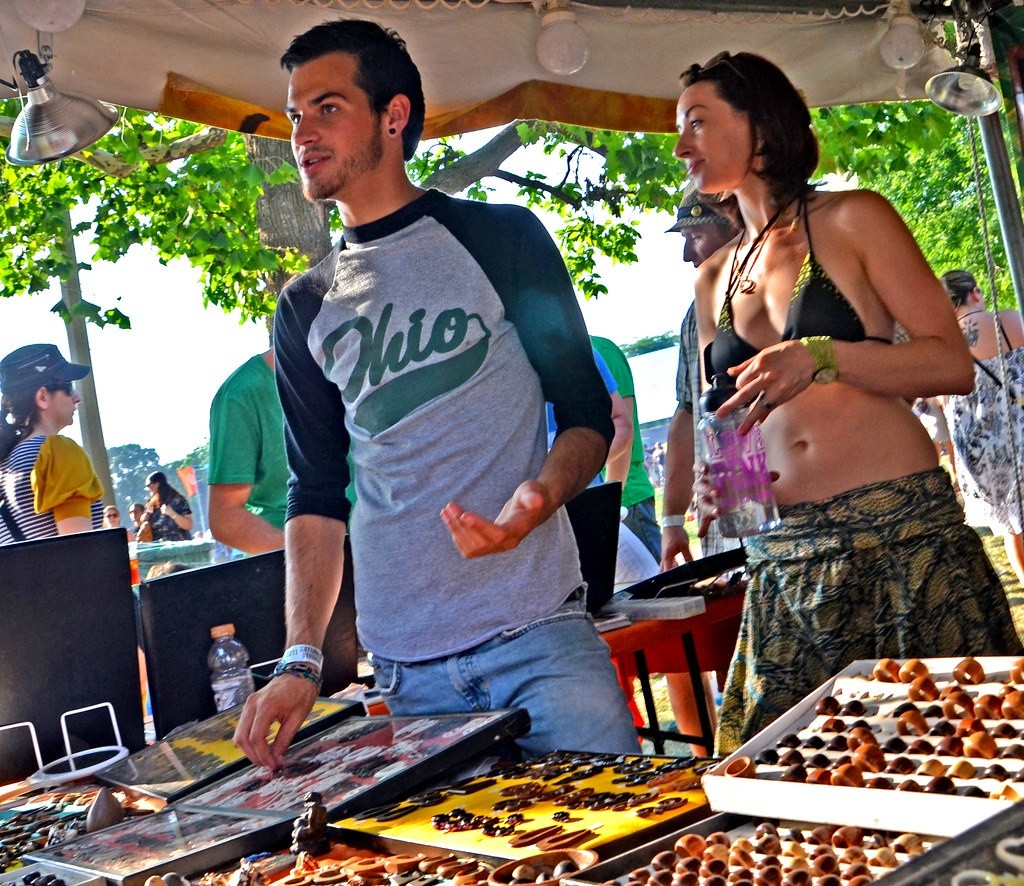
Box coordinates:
[914,270,1024,586]
[672,49,1024,752]
[104,471,193,541]
[0,344,105,543]
[207,272,357,557]
[659,178,747,572]
[547,335,662,601]
[232,18,650,768]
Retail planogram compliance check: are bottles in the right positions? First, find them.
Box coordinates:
[207,623,255,715]
[697,374,780,538]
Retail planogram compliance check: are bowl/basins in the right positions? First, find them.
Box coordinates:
[487,849,598,886]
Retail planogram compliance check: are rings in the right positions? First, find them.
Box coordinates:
[605,657,1024,886]
[762,398,772,408]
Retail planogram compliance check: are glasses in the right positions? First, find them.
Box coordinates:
[42,380,74,398]
[146,482,153,489]
[105,513,119,518]
[129,510,143,516]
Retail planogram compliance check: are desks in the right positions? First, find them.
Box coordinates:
[601,594,747,757]
[130,539,216,578]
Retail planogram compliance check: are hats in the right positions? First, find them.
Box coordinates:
[662,176,730,233]
[0,343,90,394]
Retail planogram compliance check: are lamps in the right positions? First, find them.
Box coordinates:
[925,10,1003,118]
[4,44,119,167]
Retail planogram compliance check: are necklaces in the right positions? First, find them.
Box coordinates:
[736,196,790,293]
[957,310,982,322]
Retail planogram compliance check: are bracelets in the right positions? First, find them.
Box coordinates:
[172,513,177,519]
[801,336,839,385]
[283,644,324,672]
[663,514,685,527]
[265,664,323,691]
